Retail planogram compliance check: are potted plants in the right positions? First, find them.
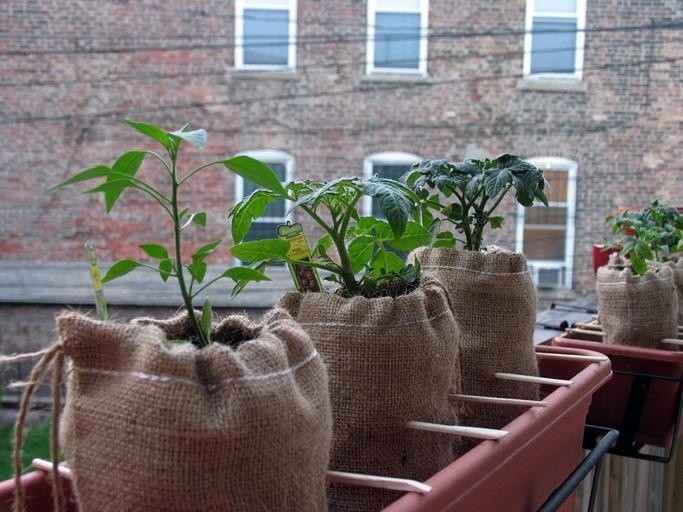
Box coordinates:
[596,202,683,349]
[231,174,461,511]
[398,153,553,438]
[45,115,330,512]
